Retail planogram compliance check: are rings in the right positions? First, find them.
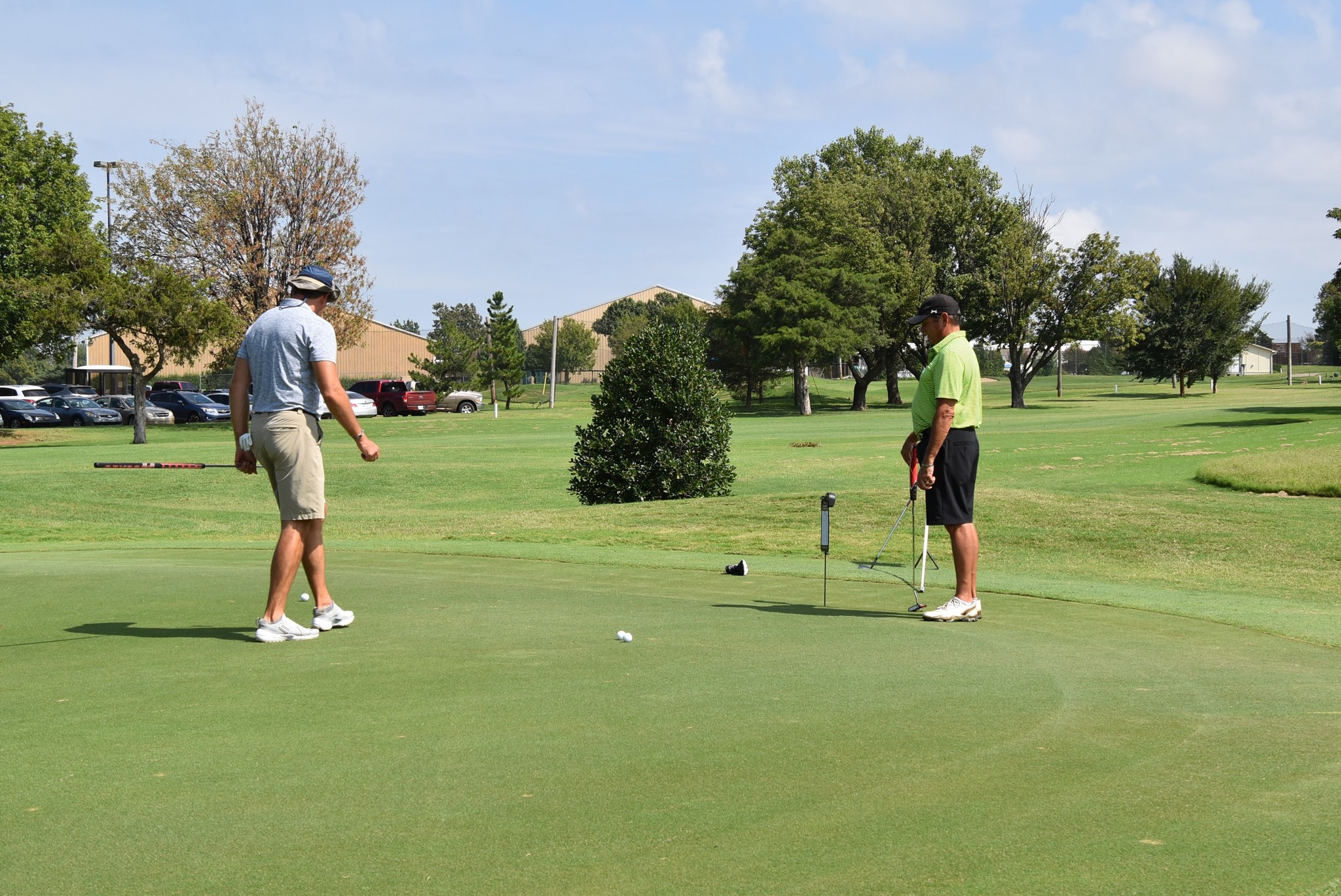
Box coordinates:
[925,480,929,484]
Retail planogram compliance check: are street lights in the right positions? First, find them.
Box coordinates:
[92,160,124,395]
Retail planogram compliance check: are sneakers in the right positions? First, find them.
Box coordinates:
[311,600,354,630]
[922,597,982,622]
[255,612,320,643]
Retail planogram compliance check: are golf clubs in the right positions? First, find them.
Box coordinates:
[908,445,928,611]
[94,462,263,469]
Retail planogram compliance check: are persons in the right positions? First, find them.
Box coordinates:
[230,266,380,642]
[901,294,982,622]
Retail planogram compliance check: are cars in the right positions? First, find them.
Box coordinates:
[0,395,175,426]
[319,391,378,419]
[406,381,483,413]
[202,387,253,422]
[122,384,152,395]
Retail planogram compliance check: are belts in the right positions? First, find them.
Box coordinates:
[916,427,931,441]
[255,408,317,419]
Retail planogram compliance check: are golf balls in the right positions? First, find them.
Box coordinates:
[623,633,632,642]
[301,593,309,601]
[617,631,625,640]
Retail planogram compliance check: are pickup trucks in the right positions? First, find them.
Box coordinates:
[347,379,437,417]
[145,380,198,398]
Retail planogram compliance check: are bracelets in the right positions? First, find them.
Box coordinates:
[353,429,364,440]
[921,464,934,467]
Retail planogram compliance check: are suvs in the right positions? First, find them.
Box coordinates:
[146,389,231,423]
[0,382,101,403]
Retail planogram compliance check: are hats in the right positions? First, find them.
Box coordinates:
[906,293,961,325]
[279,265,340,309]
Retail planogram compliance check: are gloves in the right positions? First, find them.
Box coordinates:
[239,433,253,452]
